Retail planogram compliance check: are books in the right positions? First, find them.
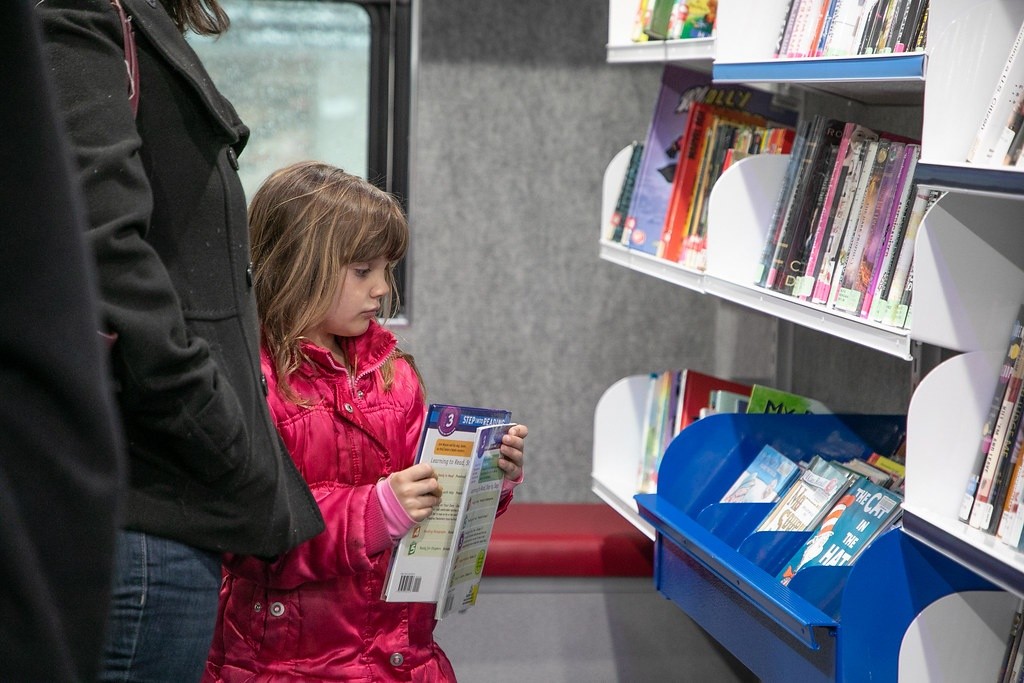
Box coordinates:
[607,1,1024,683]
[435,423,516,620]
[379,404,513,603]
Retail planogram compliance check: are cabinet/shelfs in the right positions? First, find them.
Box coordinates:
[585,0,1024,683]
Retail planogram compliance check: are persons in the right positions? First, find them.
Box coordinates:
[198,159,531,683]
[2,2,325,683]
